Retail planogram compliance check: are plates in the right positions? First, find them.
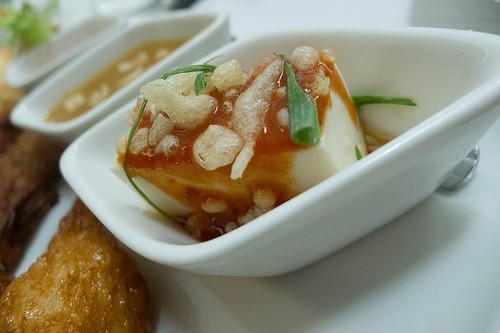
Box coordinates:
[13,1,498,332]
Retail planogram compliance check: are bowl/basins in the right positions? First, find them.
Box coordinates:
[9,10,229,146]
[60,23,500,278]
[4,14,129,93]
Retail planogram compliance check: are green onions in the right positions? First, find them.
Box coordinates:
[280,54,319,146]
